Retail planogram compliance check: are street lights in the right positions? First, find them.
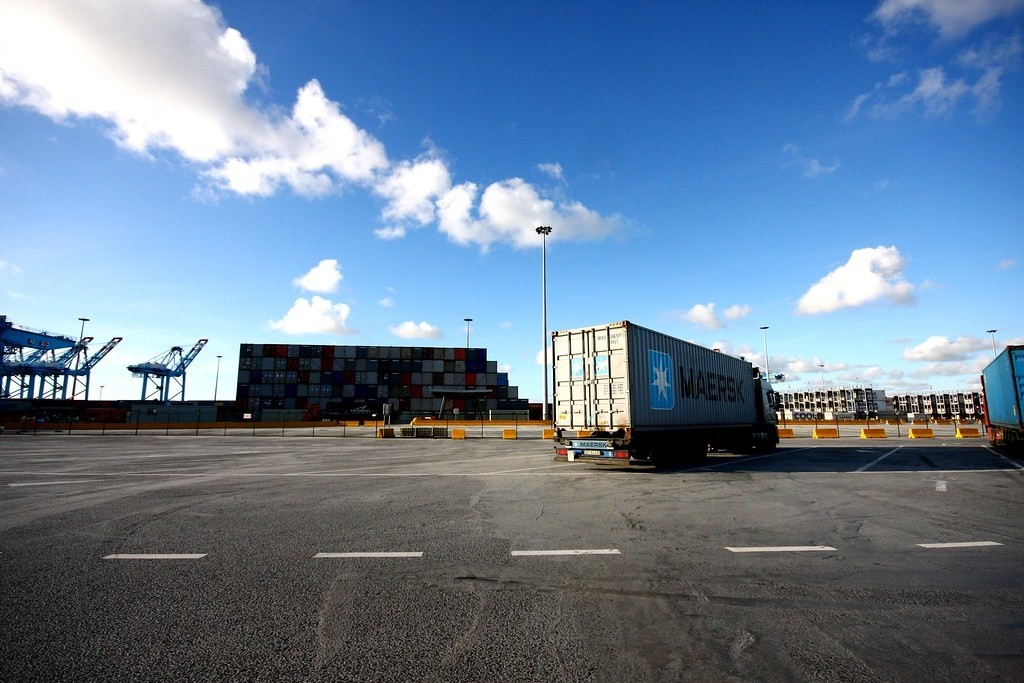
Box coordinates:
[536,226,553,420]
[819,365,824,391]
[72,317,89,399]
[464,318,473,348]
[760,327,769,383]
[214,356,223,401]
[987,329,997,359]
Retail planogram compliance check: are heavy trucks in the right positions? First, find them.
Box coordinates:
[304,403,362,422]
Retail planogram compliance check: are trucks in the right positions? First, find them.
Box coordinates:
[551,320,781,465]
[980,345,1024,450]
